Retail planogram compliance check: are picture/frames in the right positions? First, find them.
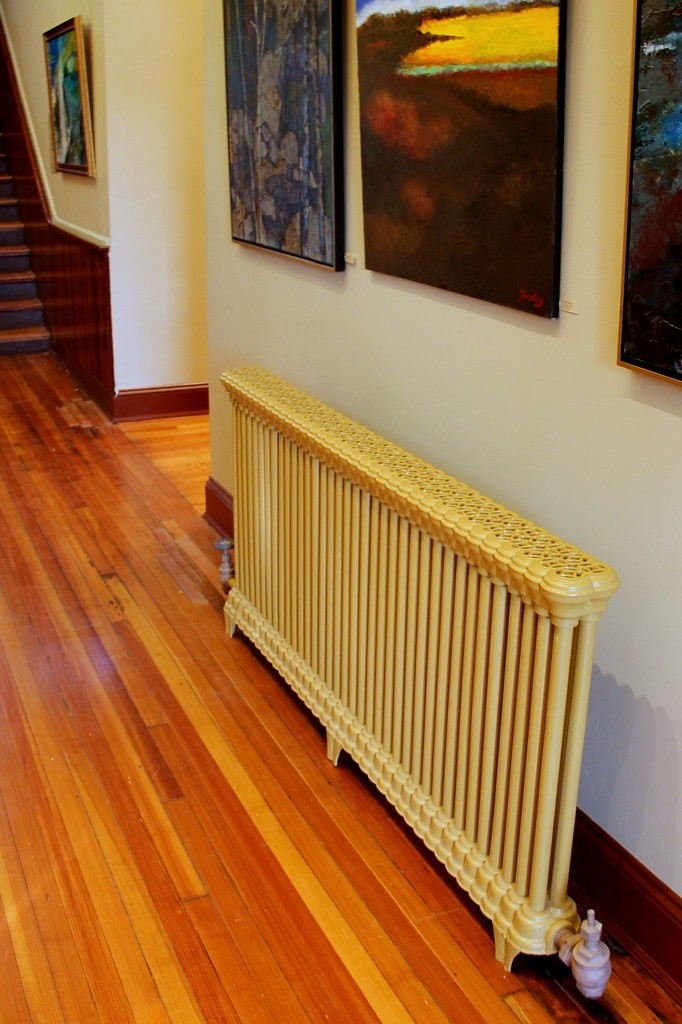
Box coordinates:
[42,15,98,180]
[222,0,347,273]
[616,0,682,387]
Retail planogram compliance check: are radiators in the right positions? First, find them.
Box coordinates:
[213,359,613,997]
